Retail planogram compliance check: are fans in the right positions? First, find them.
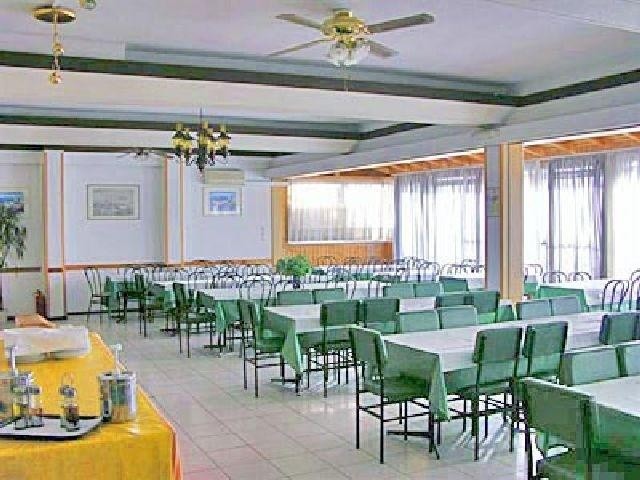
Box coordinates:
[265,8,435,58]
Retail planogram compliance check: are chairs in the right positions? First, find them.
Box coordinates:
[395,309,441,335]
[549,296,582,317]
[514,376,639,479]
[84,256,346,400]
[618,341,640,376]
[562,346,620,386]
[515,299,552,320]
[599,311,638,346]
[436,325,523,461]
[346,255,514,326]
[437,305,478,330]
[484,320,569,451]
[524,256,640,312]
[348,327,434,466]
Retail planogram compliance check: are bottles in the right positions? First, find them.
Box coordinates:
[11,371,80,432]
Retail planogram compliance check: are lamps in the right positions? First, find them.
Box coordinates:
[320,8,370,67]
[171,108,231,173]
[34,7,77,84]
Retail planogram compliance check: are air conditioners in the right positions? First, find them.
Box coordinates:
[205,169,245,186]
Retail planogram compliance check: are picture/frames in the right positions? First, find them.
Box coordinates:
[202,187,243,216]
[87,184,139,220]
[0,185,33,219]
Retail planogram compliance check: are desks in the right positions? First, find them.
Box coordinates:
[566,375,639,479]
[377,311,614,454]
[0,329,176,480]
